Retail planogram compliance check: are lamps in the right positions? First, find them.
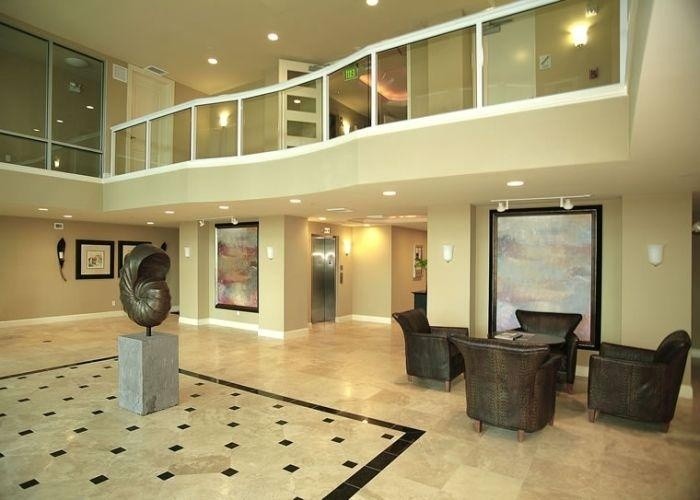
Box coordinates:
[570,21,589,49]
[443,244,453,263]
[490,194,591,213]
[183,246,191,258]
[196,216,240,227]
[265,246,274,262]
[63,57,89,68]
[646,244,663,267]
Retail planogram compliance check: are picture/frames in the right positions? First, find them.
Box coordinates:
[75,239,114,279]
[488,204,603,351]
[117,240,152,278]
[412,244,424,279]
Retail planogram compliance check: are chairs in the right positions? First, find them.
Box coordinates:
[586,329,692,432]
[392,308,469,392]
[512,309,582,394]
[448,332,564,443]
